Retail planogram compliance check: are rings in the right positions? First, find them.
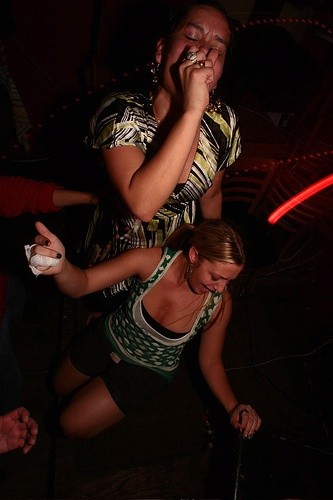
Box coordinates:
[185,50,206,69]
[238,409,249,415]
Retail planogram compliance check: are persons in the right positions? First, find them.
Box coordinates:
[62,1,243,317]
[0,179,263,455]
